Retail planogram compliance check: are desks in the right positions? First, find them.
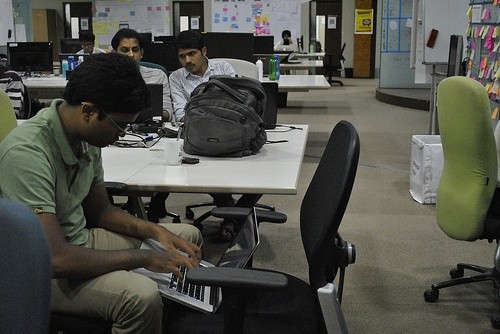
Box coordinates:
[23,74,331,98]
[131,123,310,211]
[292,52,326,57]
[277,59,325,70]
[17,120,184,203]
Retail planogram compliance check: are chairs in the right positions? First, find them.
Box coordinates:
[186,58,274,230]
[0,92,115,334]
[423,75,500,329]
[323,41,346,86]
[0,196,53,333]
[163,119,361,334]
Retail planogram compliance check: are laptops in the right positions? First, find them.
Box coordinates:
[8,41,61,74]
[260,82,278,130]
[128,206,262,314]
[131,82,163,131]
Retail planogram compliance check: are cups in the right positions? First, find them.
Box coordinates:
[165,138,184,165]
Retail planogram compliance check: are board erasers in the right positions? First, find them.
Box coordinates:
[426,29,439,48]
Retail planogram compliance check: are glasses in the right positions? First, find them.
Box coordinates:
[101,109,132,135]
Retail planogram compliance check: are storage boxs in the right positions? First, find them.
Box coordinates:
[410,133,445,204]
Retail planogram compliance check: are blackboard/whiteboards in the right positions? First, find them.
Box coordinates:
[422,1,470,64]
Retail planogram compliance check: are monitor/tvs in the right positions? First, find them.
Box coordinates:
[58,53,90,65]
[447,34,463,78]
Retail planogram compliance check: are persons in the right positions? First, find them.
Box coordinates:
[110,28,174,217]
[169,29,243,201]
[0,52,203,334]
[72,31,107,66]
[276,30,295,49]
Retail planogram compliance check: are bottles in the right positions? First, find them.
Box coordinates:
[256,57,263,81]
[62,56,84,78]
[269,55,280,80]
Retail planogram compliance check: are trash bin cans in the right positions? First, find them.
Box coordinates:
[410,135,446,204]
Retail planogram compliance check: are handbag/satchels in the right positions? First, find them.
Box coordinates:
[181,79,268,158]
